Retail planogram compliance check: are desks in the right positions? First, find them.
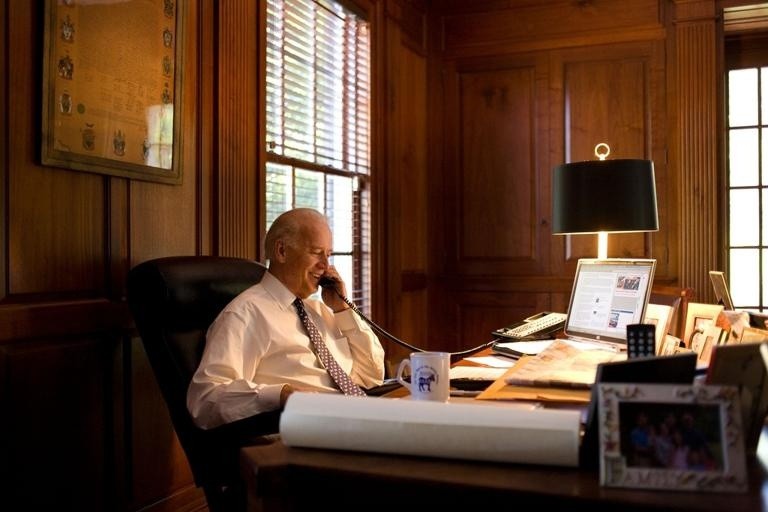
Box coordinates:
[242,306,767,510]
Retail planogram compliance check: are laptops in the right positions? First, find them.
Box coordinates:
[491,258,658,360]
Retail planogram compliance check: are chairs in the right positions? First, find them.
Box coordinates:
[129,257,390,511]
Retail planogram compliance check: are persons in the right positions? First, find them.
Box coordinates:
[186,209,386,431]
[632,411,719,473]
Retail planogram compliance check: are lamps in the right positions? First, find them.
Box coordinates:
[553,144,660,258]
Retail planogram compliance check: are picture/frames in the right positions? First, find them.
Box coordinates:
[32,1,188,185]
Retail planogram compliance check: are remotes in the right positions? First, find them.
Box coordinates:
[627,324,655,359]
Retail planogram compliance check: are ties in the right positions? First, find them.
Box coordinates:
[292,298,367,396]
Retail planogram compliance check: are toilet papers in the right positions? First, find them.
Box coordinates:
[279,391,581,468]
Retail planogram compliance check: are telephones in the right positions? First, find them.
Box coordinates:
[318,276,337,294]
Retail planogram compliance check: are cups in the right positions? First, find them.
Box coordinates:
[396,351,451,403]
